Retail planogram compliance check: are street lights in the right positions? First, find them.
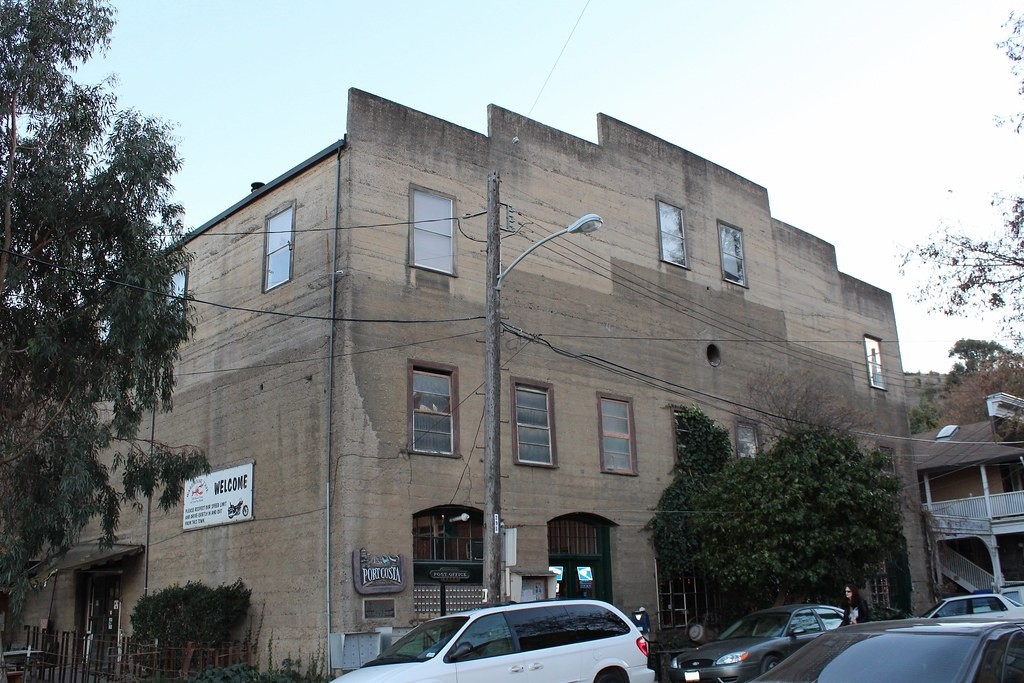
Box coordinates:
[482,172,604,600]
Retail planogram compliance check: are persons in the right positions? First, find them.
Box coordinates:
[838,584,869,627]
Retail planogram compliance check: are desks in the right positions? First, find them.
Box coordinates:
[3,650,45,670]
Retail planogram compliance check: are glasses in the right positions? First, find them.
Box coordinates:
[845,590,851,593]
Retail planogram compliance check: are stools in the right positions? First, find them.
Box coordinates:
[6,670,24,683]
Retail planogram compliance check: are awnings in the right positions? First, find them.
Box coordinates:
[0,540,144,594]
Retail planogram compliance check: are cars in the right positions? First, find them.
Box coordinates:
[904,595,1023,621]
[330,601,658,683]
[667,606,857,683]
[748,621,1024,683]
